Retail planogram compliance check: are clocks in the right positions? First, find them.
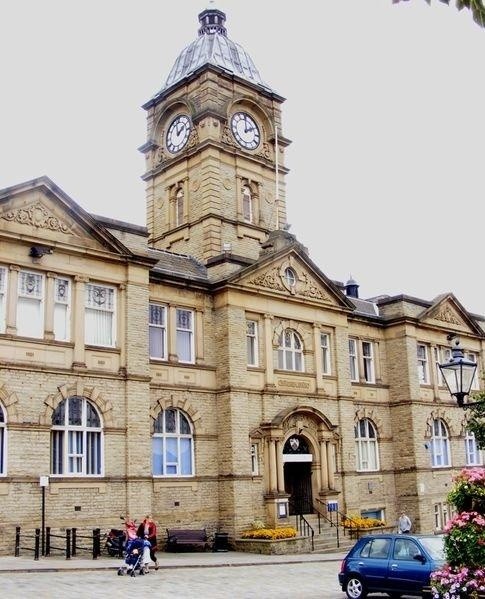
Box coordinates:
[165,113,190,153]
[230,110,262,151]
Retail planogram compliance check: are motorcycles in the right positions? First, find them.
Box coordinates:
[104,515,140,557]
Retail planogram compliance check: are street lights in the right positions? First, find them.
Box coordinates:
[40,475,50,557]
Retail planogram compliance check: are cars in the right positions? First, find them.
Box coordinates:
[339,533,452,598]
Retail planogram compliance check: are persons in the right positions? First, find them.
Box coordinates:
[136,514,159,574]
[398,510,412,534]
[121,549,139,570]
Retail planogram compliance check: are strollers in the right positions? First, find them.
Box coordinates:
[117,538,152,576]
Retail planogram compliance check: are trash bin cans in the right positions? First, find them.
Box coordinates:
[214,533,229,552]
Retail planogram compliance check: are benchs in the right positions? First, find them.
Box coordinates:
[166,528,216,553]
